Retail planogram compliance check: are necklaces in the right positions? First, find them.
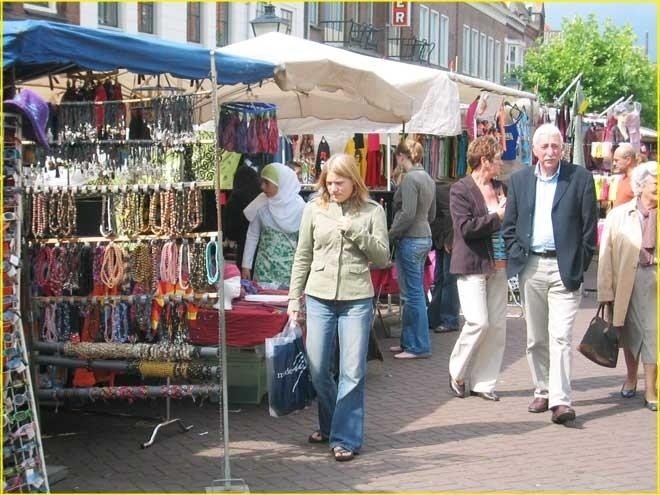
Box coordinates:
[21,186,218,344]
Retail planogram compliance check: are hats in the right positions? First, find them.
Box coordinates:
[3,89,53,146]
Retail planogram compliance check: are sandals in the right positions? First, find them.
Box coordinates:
[309,430,330,443]
[333,446,354,460]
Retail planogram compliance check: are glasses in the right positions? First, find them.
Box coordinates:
[3,110,23,305]
[3,310,47,494]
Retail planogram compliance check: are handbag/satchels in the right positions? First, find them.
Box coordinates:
[265,328,316,418]
[577,300,619,368]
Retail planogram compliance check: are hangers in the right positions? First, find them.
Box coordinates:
[493,95,529,128]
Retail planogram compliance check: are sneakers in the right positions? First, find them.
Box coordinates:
[389,345,432,359]
[434,325,458,332]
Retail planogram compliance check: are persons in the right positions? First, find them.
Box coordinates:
[393,165,459,332]
[223,163,262,266]
[597,161,656,411]
[612,145,639,207]
[504,124,599,424]
[385,140,437,359]
[288,153,393,460]
[240,161,306,290]
[448,136,507,400]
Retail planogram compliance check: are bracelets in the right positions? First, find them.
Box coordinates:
[55,340,212,406]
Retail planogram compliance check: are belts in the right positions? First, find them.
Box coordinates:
[530,250,556,257]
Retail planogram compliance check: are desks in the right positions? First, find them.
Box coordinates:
[190,289,307,405]
[368,249,437,339]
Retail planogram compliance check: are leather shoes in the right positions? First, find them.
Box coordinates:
[551,405,576,424]
[449,371,466,398]
[470,389,499,401]
[528,397,548,412]
[642,394,658,411]
[620,381,637,397]
